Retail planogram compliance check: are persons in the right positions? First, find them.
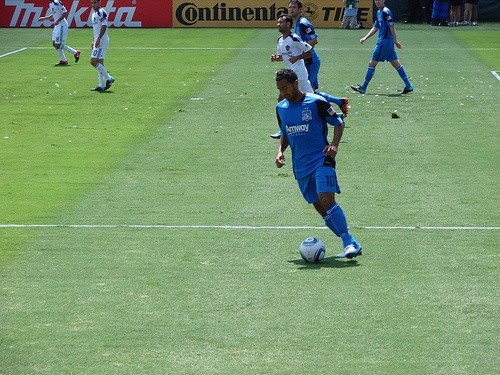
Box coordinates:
[38,0,81,66]
[274,69,363,259]
[351,0,414,95]
[404,0,478,27]
[269,0,350,138]
[89,0,116,91]
[341,0,359,29]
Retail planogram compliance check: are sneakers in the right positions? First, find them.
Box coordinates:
[90,86,105,92]
[336,244,361,257]
[75,50,80,63]
[351,84,366,94]
[56,60,68,65]
[344,246,357,259]
[105,76,115,90]
[399,85,414,94]
[339,98,348,117]
[271,131,281,139]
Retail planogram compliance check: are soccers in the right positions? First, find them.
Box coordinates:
[300,237,326,262]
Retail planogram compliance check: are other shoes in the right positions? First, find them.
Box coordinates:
[431,19,477,26]
[358,22,363,29]
